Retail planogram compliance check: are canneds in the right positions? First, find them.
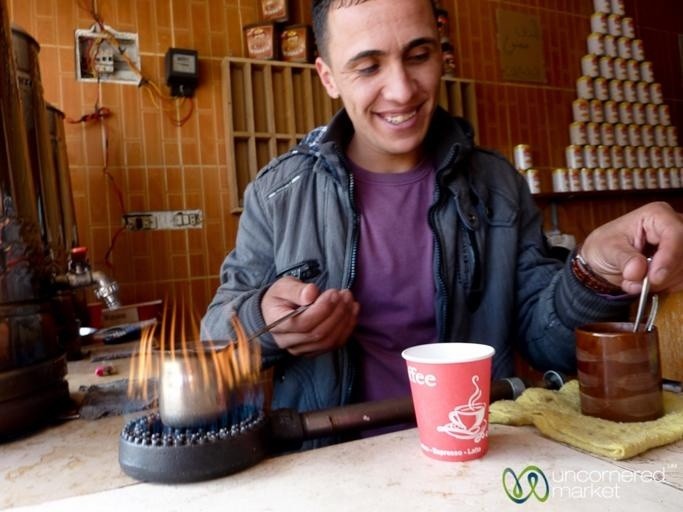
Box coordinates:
[522,169,541,194]
[512,143,532,173]
[552,1,683,193]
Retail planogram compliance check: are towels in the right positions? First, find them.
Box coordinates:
[487,374,683,457]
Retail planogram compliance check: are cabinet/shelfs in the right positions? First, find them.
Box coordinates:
[219,53,481,215]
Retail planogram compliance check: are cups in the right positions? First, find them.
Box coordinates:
[401,340,496,463]
[148,340,234,427]
[572,318,666,425]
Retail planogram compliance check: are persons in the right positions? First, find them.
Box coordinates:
[195,1,681,455]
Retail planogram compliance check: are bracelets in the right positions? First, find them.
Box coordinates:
[570,245,626,300]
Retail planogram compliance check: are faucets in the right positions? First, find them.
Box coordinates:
[52,247,123,313]
